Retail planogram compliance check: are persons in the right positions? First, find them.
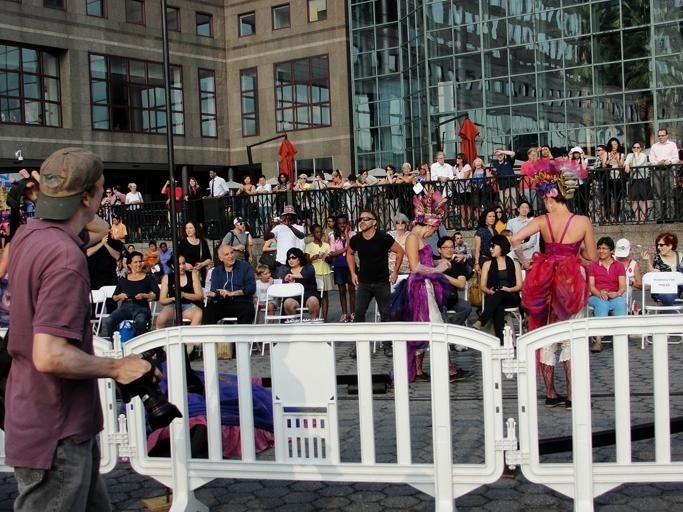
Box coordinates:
[561,151,568,160]
[569,146,590,216]
[159,242,173,273]
[625,142,650,226]
[500,230,514,261]
[472,158,485,220]
[168,218,212,288]
[346,210,404,358]
[155,253,203,357]
[594,145,607,203]
[0,147,164,512]
[487,160,499,204]
[430,151,453,228]
[253,264,278,324]
[87,236,126,334]
[506,201,537,261]
[283,247,321,323]
[330,214,357,323]
[649,127,680,225]
[262,221,277,253]
[537,144,555,161]
[494,206,506,233]
[406,190,476,384]
[142,240,159,273]
[452,232,472,281]
[510,160,596,411]
[615,238,642,311]
[115,260,126,278]
[123,245,135,271]
[412,162,430,185]
[221,216,252,264]
[385,212,410,292]
[519,147,537,216]
[305,224,334,322]
[602,137,625,223]
[434,235,472,351]
[379,164,398,231]
[473,235,522,346]
[102,251,160,346]
[640,231,683,308]
[101,169,378,221]
[593,236,626,353]
[264,205,305,307]
[395,163,414,220]
[325,216,335,243]
[202,243,256,335]
[474,208,498,273]
[489,148,516,219]
[453,153,472,229]
[109,214,128,244]
[1,240,11,325]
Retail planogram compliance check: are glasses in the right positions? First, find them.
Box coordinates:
[496,211,502,213]
[656,243,667,246]
[658,135,666,137]
[106,192,111,193]
[396,220,406,224]
[441,245,455,250]
[488,244,496,248]
[596,150,600,151]
[358,217,376,222]
[288,256,297,259]
[456,157,462,159]
[237,222,245,225]
[633,147,639,148]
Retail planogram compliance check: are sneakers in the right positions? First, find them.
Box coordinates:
[450,368,474,382]
[350,313,355,322]
[565,400,594,410]
[338,314,349,323]
[545,394,567,407]
[591,341,603,352]
[384,345,393,357]
[350,345,357,357]
[414,373,430,381]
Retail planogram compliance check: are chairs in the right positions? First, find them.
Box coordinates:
[480,268,525,343]
[200,280,260,358]
[640,271,683,350]
[587,267,628,345]
[256,279,283,325]
[295,278,325,321]
[261,282,304,357]
[373,274,409,354]
[95,284,117,340]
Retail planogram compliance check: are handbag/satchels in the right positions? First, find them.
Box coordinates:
[579,158,593,185]
[594,151,612,180]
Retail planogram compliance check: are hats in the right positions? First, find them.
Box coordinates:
[298,173,307,178]
[33,147,104,220]
[615,238,630,257]
[233,216,247,225]
[281,205,296,215]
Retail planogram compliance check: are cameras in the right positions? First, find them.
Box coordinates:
[117,363,183,432]
